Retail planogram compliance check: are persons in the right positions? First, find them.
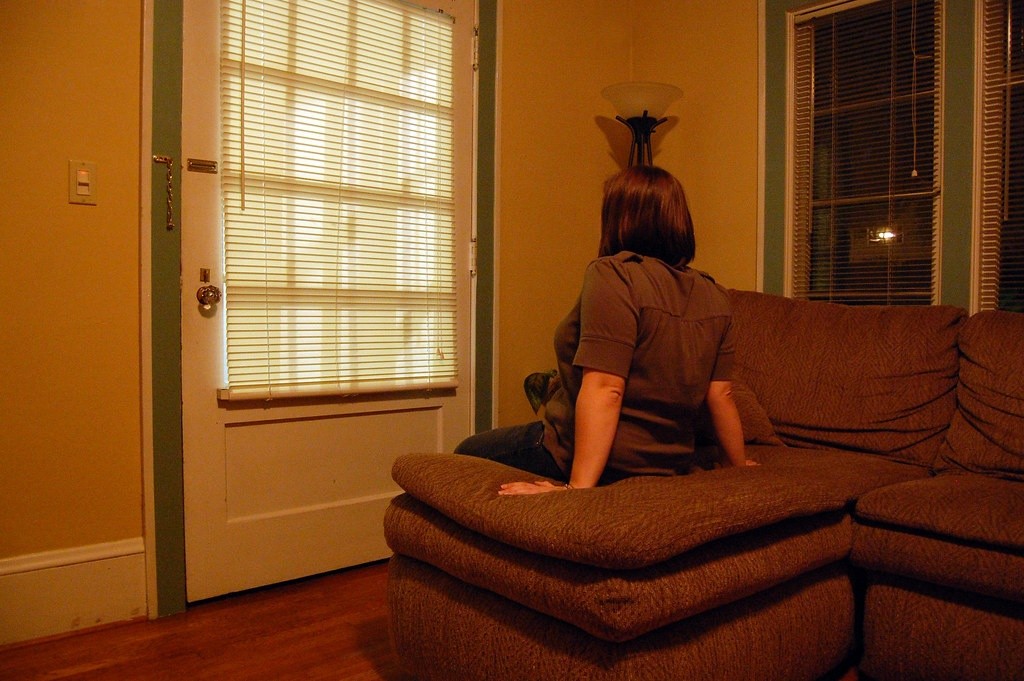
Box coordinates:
[454,164,763,495]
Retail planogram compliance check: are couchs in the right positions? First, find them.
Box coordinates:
[381,288,1024,681]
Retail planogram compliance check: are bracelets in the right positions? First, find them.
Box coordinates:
[565,484,574,489]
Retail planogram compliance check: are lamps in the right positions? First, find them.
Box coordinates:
[601,80,680,167]
